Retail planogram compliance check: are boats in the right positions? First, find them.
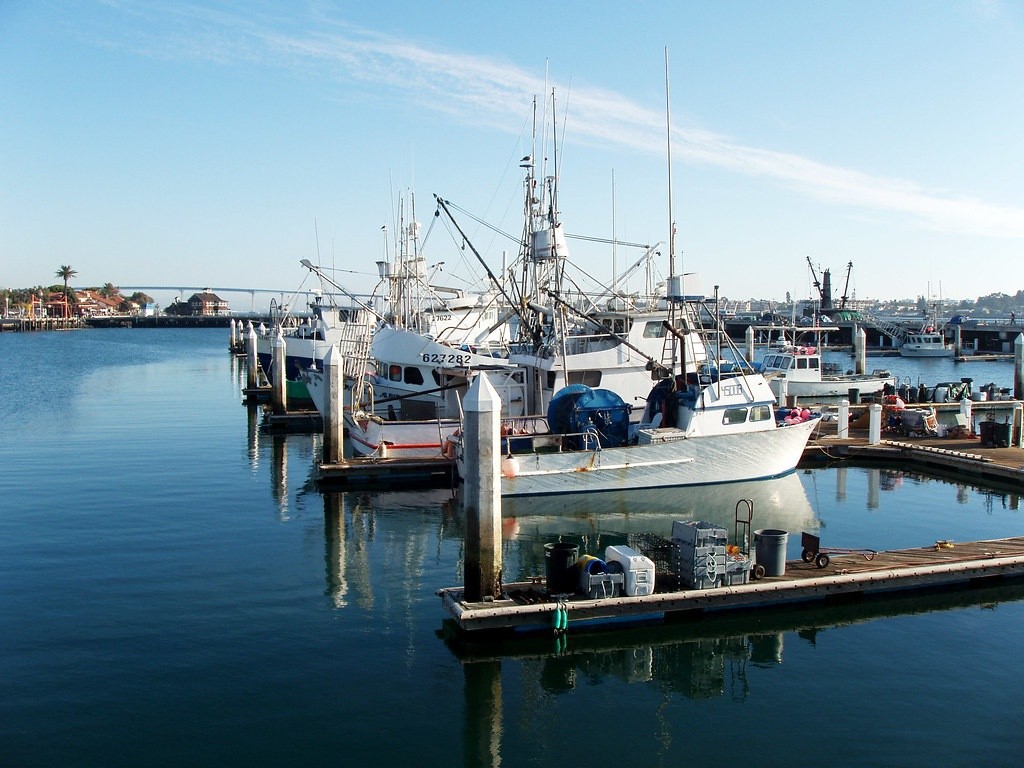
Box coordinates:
[757,344,898,399]
[250,55,713,461]
[454,46,827,494]
[898,328,960,357]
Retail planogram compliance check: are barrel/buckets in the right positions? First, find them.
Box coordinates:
[544,543,580,591]
[578,554,607,575]
[979,421,996,446]
[935,386,948,402]
[753,529,789,576]
[848,388,859,404]
[785,394,798,408]
[994,423,1012,448]
[926,387,936,401]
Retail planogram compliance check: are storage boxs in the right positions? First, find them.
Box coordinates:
[670,520,754,590]
[638,427,685,447]
[576,571,625,599]
